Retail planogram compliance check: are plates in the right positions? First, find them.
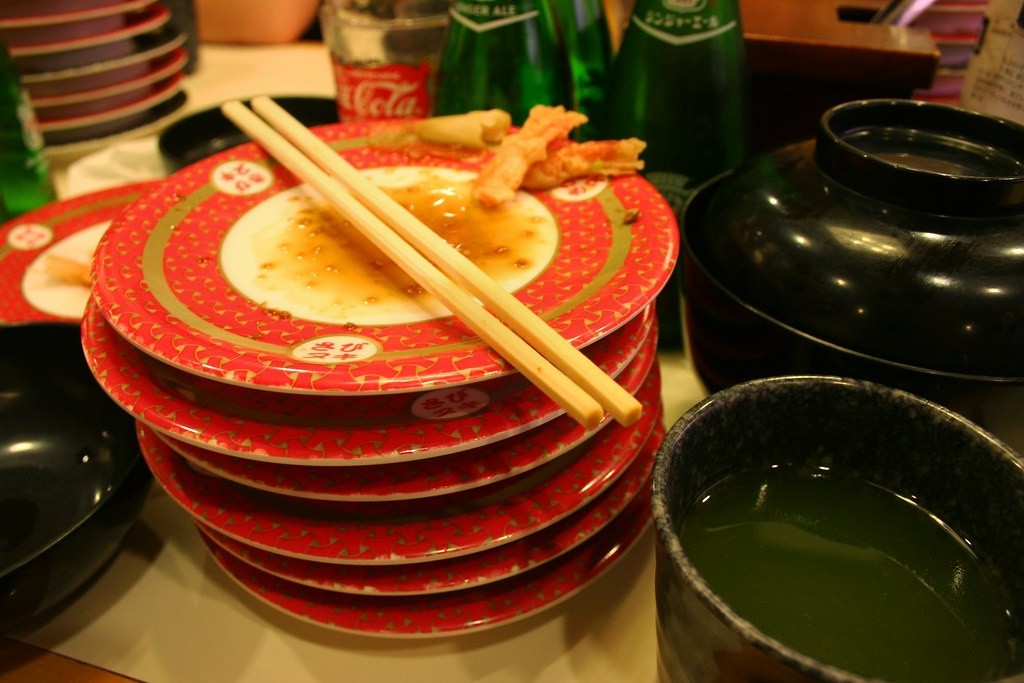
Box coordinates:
[0,0,191,163]
[0,179,166,321]
[80,114,681,640]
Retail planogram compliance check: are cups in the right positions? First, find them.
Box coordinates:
[650,373,1024,683]
[319,0,452,121]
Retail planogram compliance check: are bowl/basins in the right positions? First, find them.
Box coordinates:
[0,321,152,645]
[682,98,1024,455]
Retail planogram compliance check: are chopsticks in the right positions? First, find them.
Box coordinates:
[223,94,643,429]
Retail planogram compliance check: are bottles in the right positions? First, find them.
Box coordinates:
[433,0,752,179]
[0,33,58,217]
